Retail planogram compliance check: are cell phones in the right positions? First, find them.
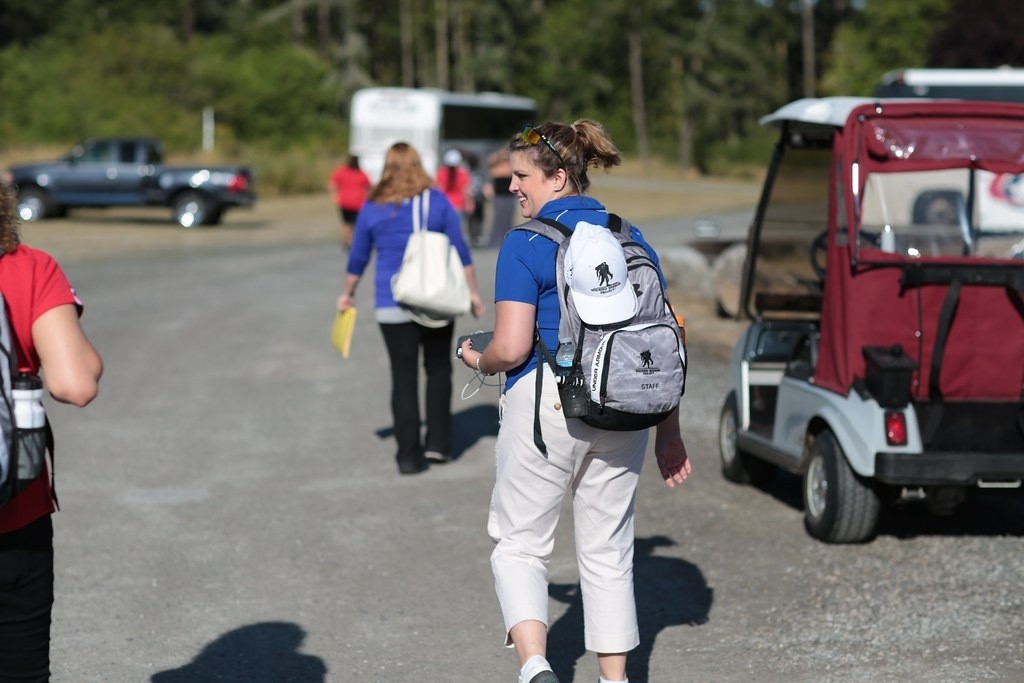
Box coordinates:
[456,331,495,359]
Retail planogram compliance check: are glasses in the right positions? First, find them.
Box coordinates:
[521,125,567,171]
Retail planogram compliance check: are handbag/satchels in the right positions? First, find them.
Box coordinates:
[388,189,470,328]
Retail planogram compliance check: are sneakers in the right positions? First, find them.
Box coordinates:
[424,448,455,461]
[518,656,560,683]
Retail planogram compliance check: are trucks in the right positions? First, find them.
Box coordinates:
[346,85,539,222]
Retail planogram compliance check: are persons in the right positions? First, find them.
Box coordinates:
[336,143,485,475]
[329,154,375,249]
[455,117,693,683]
[435,148,475,242]
[461,153,495,250]
[0,171,104,683]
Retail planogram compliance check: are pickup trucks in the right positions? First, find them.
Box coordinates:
[0,133,259,228]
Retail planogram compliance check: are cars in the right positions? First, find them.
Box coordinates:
[870,65,1024,103]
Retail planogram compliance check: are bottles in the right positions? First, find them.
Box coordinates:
[11,367,46,480]
[557,335,589,418]
[672,305,685,347]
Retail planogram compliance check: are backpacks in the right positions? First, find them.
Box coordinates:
[0,288,49,512]
[506,213,689,434]
